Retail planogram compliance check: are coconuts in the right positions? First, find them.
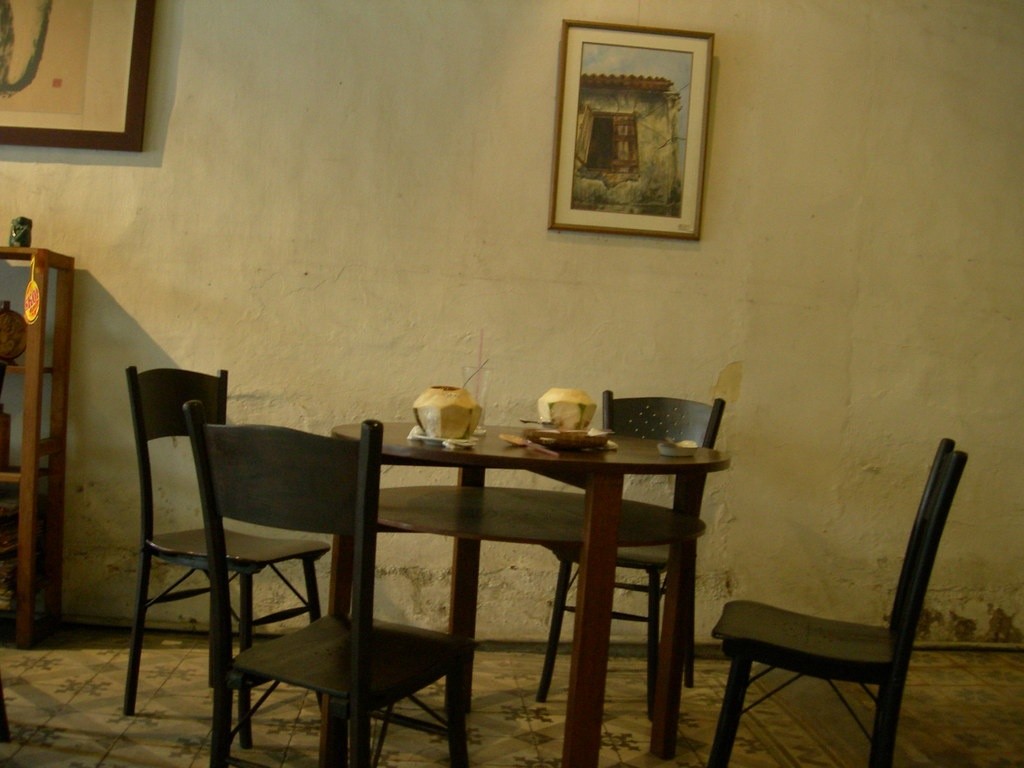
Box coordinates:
[538,388,597,430]
[414,385,483,439]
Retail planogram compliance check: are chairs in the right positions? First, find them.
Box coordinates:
[327,421,733,768]
[706,436,967,768]
[536,390,729,723]
[181,399,478,768]
[124,364,331,750]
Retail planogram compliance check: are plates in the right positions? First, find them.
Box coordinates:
[413,432,479,447]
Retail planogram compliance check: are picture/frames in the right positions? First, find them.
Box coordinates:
[1,0,157,153]
[547,19,715,243]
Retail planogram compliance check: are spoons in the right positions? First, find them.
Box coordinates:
[499,434,559,456]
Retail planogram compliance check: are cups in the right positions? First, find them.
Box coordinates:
[9,216,33,247]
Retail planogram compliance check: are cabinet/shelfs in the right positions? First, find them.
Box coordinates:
[0,247,76,651]
